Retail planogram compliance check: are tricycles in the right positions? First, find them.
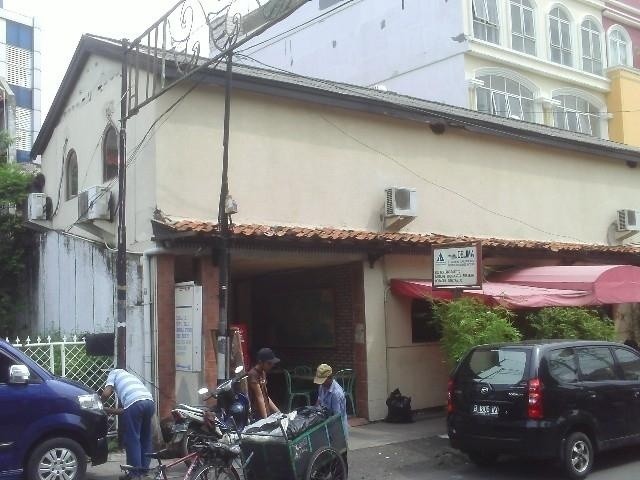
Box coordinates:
[190,401,354,480]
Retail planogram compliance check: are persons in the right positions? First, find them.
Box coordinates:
[313,363,349,474]
[249,346,280,421]
[102,369,156,480]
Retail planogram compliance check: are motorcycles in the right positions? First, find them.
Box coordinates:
[175,362,254,469]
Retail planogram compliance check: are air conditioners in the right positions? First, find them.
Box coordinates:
[383,184,421,218]
[75,183,114,225]
[26,191,50,222]
[616,207,640,232]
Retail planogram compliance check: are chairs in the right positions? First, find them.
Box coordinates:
[281,369,317,411]
[334,366,358,417]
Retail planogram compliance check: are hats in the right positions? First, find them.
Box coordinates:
[258,348,280,363]
[313,364,332,385]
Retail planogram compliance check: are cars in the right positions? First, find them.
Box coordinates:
[0,336,112,480]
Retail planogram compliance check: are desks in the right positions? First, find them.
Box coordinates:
[294,366,350,382]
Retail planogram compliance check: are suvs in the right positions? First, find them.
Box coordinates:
[445,336,639,480]
